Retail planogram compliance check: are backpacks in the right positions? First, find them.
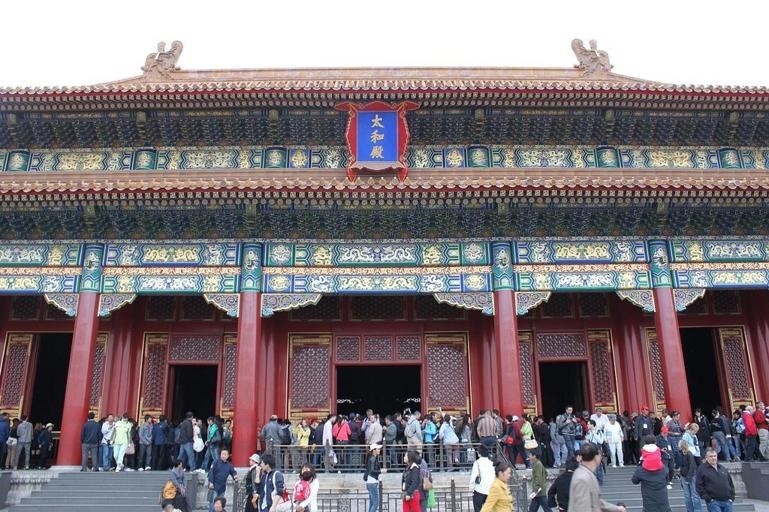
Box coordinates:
[575,419,583,439]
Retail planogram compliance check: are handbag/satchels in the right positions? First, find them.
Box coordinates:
[524,438,538,449]
[163,481,177,499]
[423,477,433,491]
[431,432,440,443]
[428,489,436,509]
[271,487,292,512]
[126,442,136,455]
[193,438,204,452]
[6,437,17,446]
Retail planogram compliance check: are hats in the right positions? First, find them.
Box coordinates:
[249,454,261,464]
[45,423,55,429]
[369,443,383,451]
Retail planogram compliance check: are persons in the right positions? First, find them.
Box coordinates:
[161,450,320,512]
[261,402,769,512]
[80,412,232,474]
[0,413,54,470]
